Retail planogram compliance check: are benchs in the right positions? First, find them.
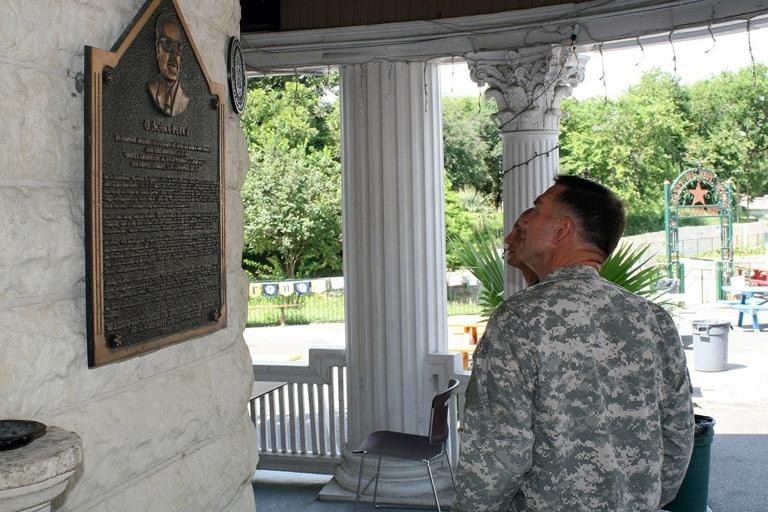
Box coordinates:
[245,304,299,327]
[447,330,485,367]
[718,299,768,333]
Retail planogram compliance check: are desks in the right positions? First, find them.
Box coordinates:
[448,322,486,346]
[722,286,768,333]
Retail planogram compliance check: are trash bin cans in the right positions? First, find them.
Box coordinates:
[692,319,733,372]
[662,415,715,512]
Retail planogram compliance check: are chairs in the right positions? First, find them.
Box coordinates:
[351,376,461,512]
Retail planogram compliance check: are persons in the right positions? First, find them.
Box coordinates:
[147,13,190,116]
[452,175,696,511]
